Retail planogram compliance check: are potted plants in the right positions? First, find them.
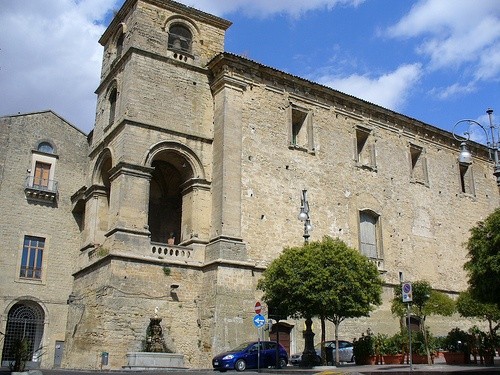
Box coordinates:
[441,327,474,365]
[353,329,440,365]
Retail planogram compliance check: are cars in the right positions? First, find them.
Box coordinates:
[288,339,356,366]
[212,340,289,373]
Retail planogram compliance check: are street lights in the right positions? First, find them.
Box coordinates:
[451,106,500,203]
[297,188,318,366]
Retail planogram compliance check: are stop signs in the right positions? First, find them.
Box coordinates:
[254,302,262,314]
